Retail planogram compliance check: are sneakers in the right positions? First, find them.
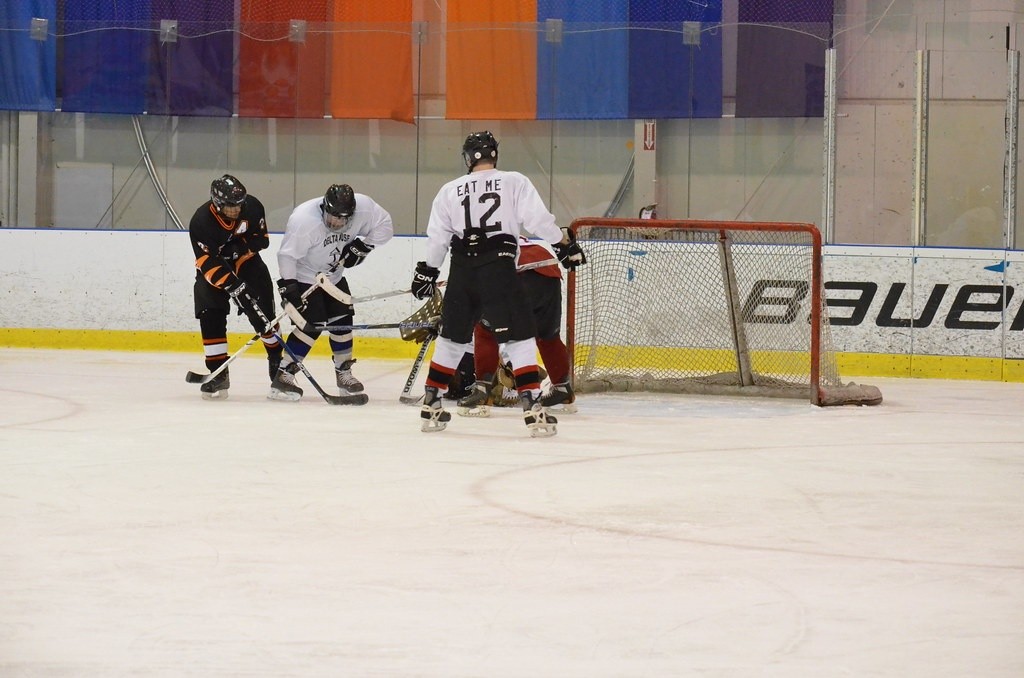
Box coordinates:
[520,390,559,437]
[200,356,231,400]
[538,380,577,413]
[457,379,493,418]
[332,354,365,397]
[268,361,303,402]
[420,385,451,432]
[268,352,283,383]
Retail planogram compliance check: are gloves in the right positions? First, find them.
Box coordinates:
[338,237,375,268]
[221,240,246,265]
[551,227,587,272]
[276,277,308,314]
[411,262,440,301]
[227,279,264,314]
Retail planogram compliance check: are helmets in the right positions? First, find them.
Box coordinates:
[462,131,497,170]
[211,174,247,220]
[324,183,355,230]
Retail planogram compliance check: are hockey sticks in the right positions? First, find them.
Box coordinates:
[315,253,582,305]
[399,328,434,406]
[220,257,370,406]
[280,297,401,333]
[184,259,346,386]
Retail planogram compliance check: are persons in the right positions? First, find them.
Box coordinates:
[189,173,286,392]
[411,131,586,428]
[271,182,393,397]
